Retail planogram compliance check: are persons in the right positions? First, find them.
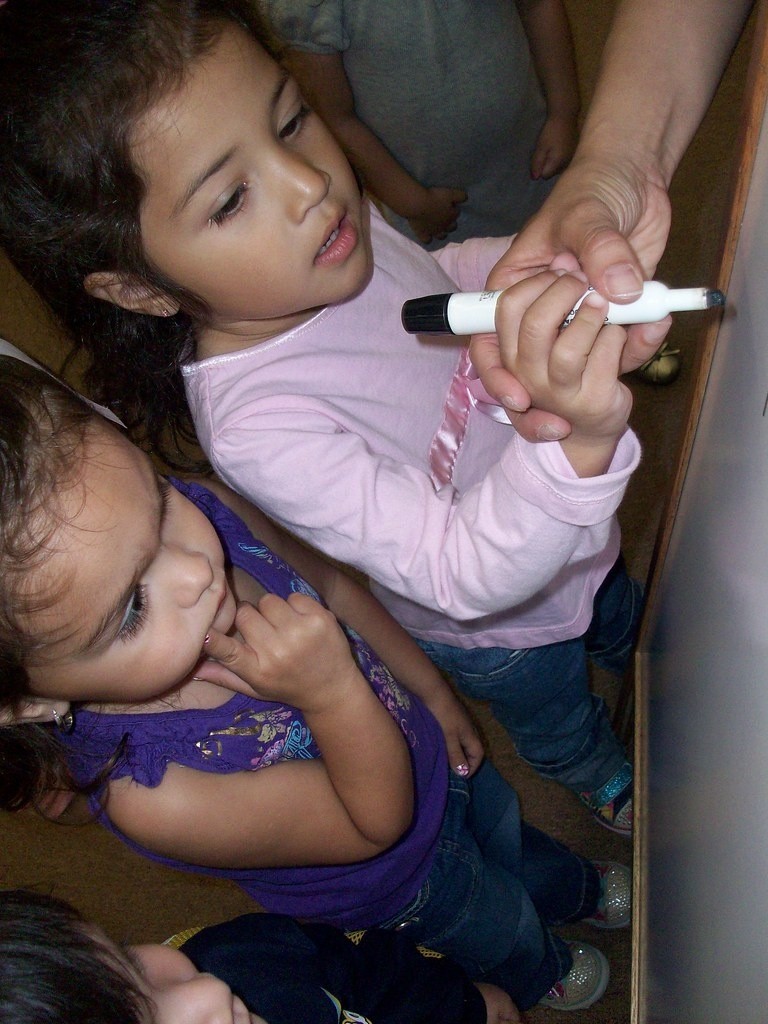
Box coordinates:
[242,0,581,255]
[0,887,523,1024]
[1,351,634,1014]
[0,0,641,838]
[468,0,750,442]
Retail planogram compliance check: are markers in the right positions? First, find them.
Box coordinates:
[400,279,727,336]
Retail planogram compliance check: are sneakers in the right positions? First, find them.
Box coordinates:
[576,758,636,836]
[536,939,607,1010]
[578,861,632,927]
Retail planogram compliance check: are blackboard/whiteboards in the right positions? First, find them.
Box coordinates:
[630,0,767,1023]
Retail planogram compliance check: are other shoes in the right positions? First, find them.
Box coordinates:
[634,341,682,383]
